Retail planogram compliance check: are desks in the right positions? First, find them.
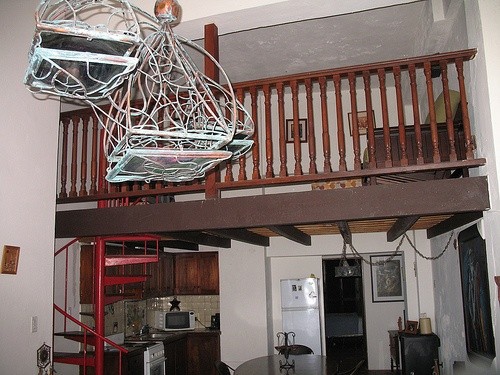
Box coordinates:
[388,330,440,375]
[234,354,339,375]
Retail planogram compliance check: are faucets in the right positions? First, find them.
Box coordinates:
[135,324,153,336]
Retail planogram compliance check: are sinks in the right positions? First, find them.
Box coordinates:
[128,331,174,340]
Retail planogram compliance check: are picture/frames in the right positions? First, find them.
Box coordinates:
[286,119,308,143]
[1,246,20,275]
[370,254,404,303]
[347,110,376,137]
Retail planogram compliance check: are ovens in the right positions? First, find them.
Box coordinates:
[144,342,168,375]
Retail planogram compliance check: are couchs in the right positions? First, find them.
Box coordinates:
[365,89,469,185]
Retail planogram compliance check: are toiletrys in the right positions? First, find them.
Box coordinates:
[419,307,430,335]
[113,321,118,333]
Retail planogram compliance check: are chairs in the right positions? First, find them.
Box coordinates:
[214,360,235,375]
[340,360,366,375]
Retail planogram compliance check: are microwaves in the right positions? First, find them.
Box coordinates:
[154,309,196,331]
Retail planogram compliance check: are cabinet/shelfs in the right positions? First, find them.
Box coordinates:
[80,244,221,305]
[163,332,220,375]
[79,345,145,375]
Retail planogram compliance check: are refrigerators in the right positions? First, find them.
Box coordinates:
[279,278,321,354]
